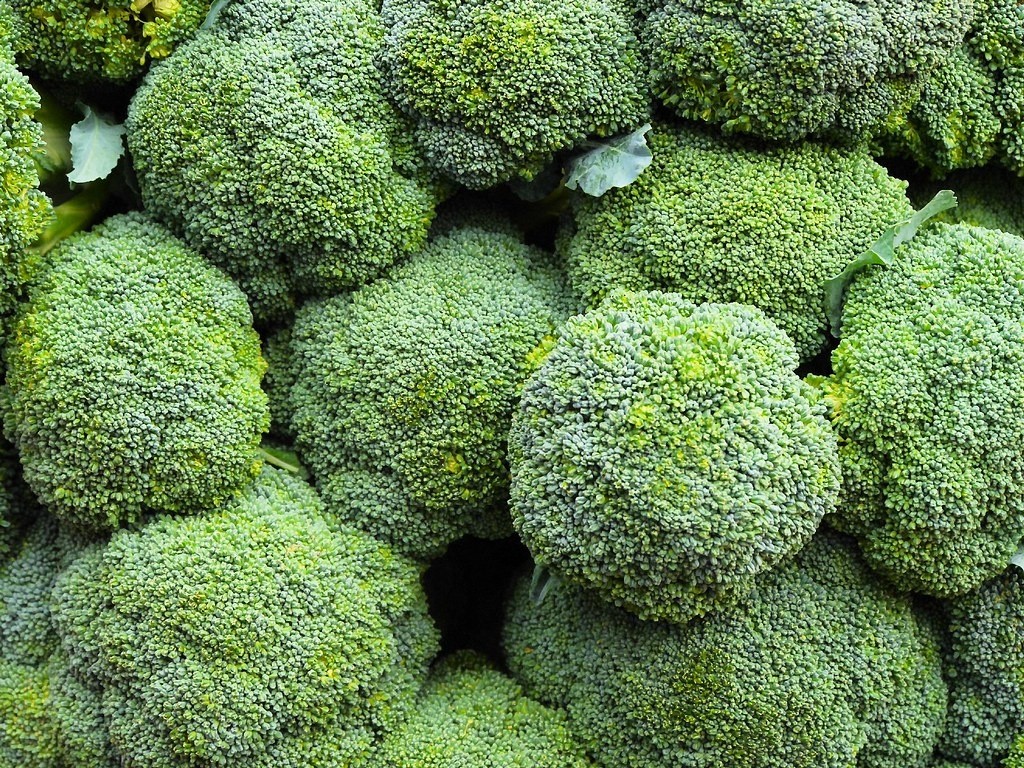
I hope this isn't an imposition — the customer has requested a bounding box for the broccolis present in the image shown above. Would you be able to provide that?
[3,0,1023,768]
